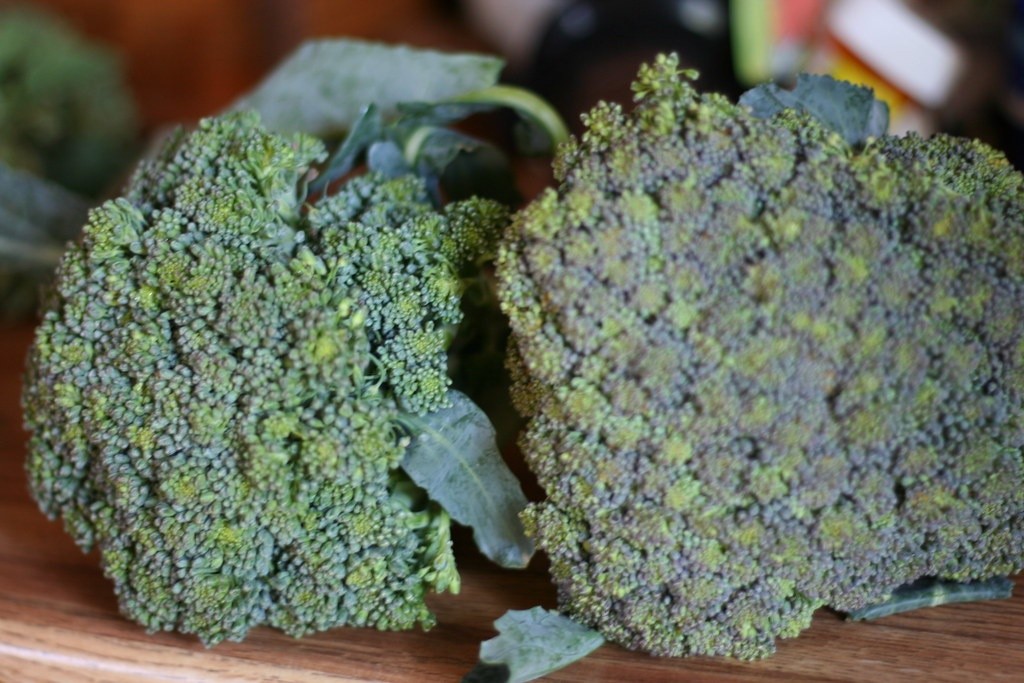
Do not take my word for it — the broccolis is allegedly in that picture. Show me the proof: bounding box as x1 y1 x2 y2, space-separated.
20 41 580 647
476 51 1024 683
